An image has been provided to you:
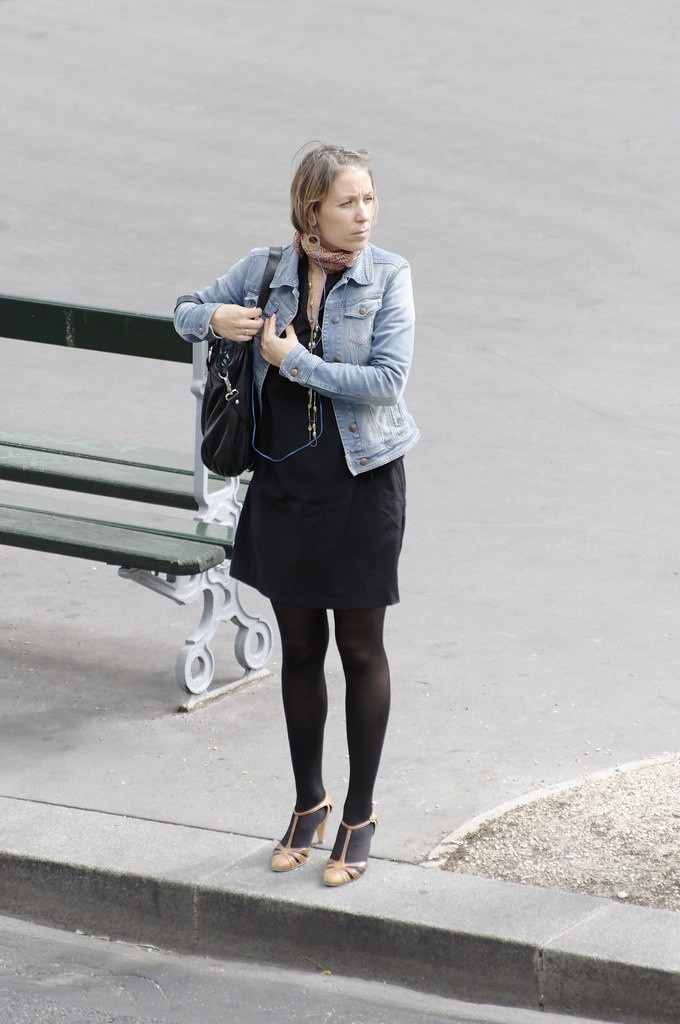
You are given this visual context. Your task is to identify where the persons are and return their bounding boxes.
[172,140,421,887]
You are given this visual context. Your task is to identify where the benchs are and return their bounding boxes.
[0,295,273,714]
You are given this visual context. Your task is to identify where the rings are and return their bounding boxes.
[246,329,248,336]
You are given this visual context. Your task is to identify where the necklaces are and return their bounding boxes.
[305,239,325,446]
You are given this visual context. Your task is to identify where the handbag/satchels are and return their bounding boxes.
[198,247,283,477]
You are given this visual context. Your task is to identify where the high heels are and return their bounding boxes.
[271,790,334,871]
[322,804,378,887]
